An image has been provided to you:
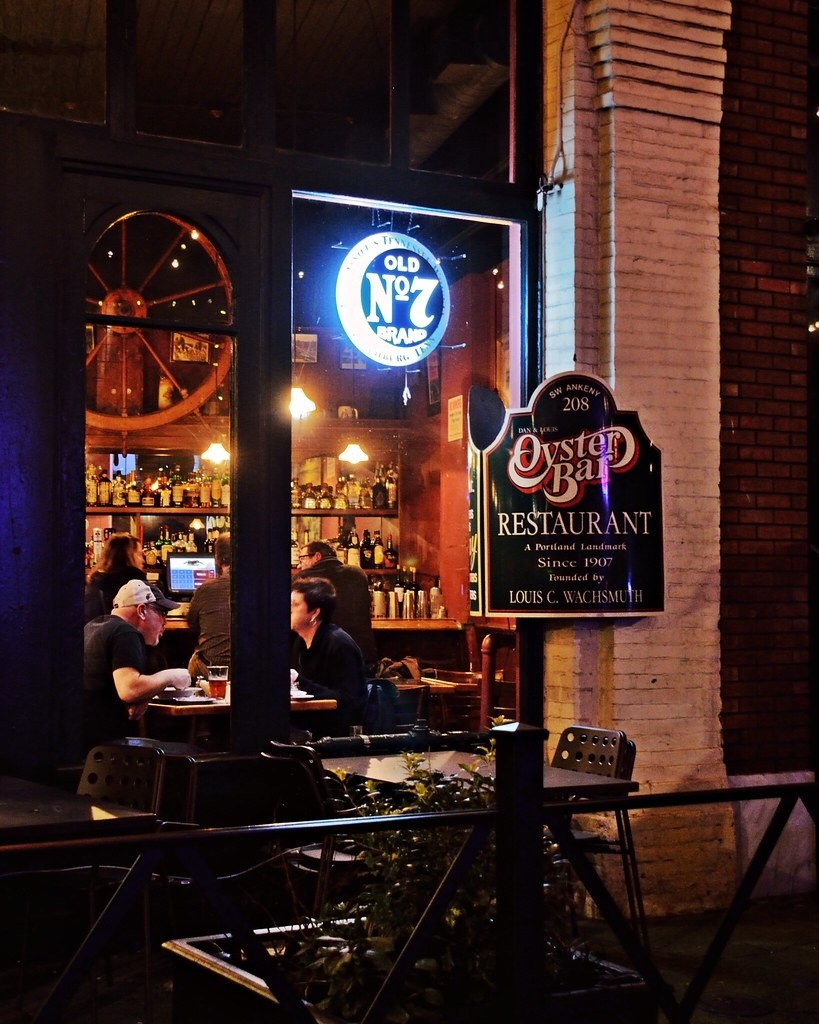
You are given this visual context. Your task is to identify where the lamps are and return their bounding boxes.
[338,346,369,465]
[200,252,230,465]
[290,291,315,418]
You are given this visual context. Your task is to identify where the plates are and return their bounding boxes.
[156,686,203,697]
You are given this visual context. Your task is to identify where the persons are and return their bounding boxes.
[291,577,367,740]
[181,531,231,681]
[293,541,371,662]
[84,579,191,738]
[84,533,145,625]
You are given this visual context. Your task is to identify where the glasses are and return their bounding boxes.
[299,554,316,564]
[147,604,168,617]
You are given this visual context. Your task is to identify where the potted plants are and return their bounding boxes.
[162,716,675,1024]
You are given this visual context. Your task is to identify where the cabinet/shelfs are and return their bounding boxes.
[86,492,400,576]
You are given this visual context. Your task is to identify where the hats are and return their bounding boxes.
[113,579,180,611]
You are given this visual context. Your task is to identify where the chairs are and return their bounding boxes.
[17,745,196,1024]
[257,740,364,921]
[549,727,650,956]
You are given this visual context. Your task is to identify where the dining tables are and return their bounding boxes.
[146,699,339,749]
[388,676,455,730]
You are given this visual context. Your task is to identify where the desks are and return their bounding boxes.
[0,777,157,1024]
[308,751,640,983]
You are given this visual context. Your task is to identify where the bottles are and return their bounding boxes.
[290,463,419,607]
[204,393,219,416]
[84,515,230,567]
[85,464,230,507]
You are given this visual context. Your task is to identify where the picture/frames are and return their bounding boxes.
[169,330,212,364]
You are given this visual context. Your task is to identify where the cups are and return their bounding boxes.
[207,665,229,699]
[338,405,359,419]
[371,587,444,618]
